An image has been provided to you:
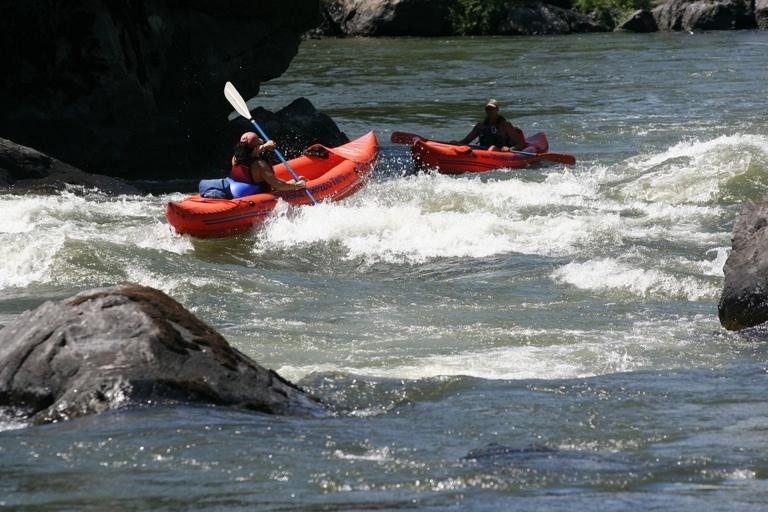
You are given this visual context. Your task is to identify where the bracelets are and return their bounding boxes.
[295,184,296,191]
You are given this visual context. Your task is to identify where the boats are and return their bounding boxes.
[410,131,548,174]
[166,130,379,238]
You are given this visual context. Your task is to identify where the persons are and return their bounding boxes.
[457,98,525,152]
[232,131,306,193]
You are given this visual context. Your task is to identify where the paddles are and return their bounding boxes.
[390,131,576,166]
[224,79,325,208]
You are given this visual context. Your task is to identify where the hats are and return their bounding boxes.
[485,98,497,108]
[240,132,263,146]
